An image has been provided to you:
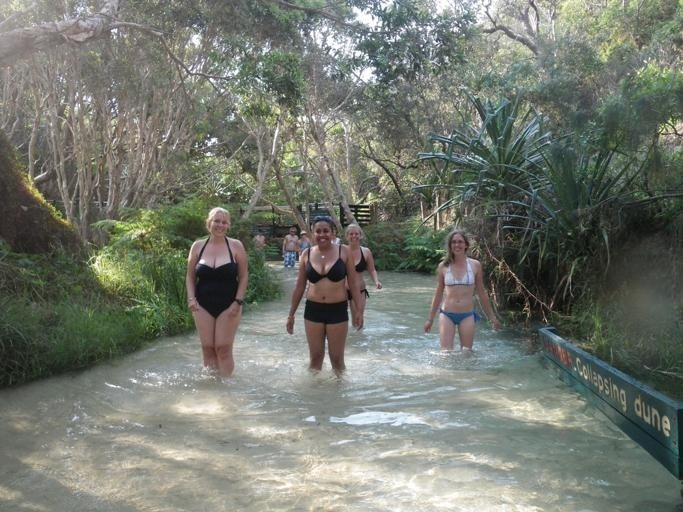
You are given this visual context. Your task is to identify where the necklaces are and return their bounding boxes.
[319,254,327,260]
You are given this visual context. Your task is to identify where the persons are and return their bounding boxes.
[183,205,249,380]
[296,229,311,261]
[281,226,300,269]
[330,229,340,246]
[422,227,502,359]
[285,213,363,382]
[251,229,266,262]
[340,222,382,332]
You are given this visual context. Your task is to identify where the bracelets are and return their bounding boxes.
[186,297,195,301]
[287,315,295,320]
[233,298,245,306]
[426,318,432,322]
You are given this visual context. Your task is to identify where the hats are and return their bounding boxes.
[300,230,307,235]
